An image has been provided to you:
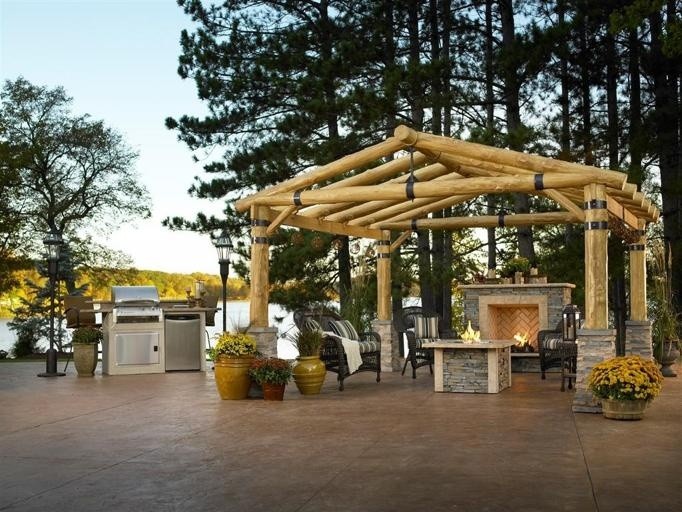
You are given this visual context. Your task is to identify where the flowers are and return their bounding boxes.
[245,356,295,388]
[206,330,262,362]
[584,353,666,404]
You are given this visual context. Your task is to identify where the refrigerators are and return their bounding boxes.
[162,314,202,373]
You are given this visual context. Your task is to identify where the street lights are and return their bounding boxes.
[212,224,234,371]
[38,222,67,377]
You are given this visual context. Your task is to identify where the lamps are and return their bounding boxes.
[559,303,582,342]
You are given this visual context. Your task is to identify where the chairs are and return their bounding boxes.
[536,302,586,380]
[293,306,382,391]
[394,304,459,380]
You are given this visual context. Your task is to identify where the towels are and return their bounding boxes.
[322,331,364,376]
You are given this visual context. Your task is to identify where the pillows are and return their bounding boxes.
[327,317,361,343]
[415,316,441,339]
[305,319,324,334]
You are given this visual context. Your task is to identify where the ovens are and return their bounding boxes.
[102,328,164,376]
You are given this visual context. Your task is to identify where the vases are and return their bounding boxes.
[212,352,253,401]
[260,381,284,401]
[597,397,647,420]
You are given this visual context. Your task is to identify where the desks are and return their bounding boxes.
[554,342,577,392]
[422,339,517,395]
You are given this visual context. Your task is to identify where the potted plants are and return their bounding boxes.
[286,326,328,395]
[70,326,103,377]
